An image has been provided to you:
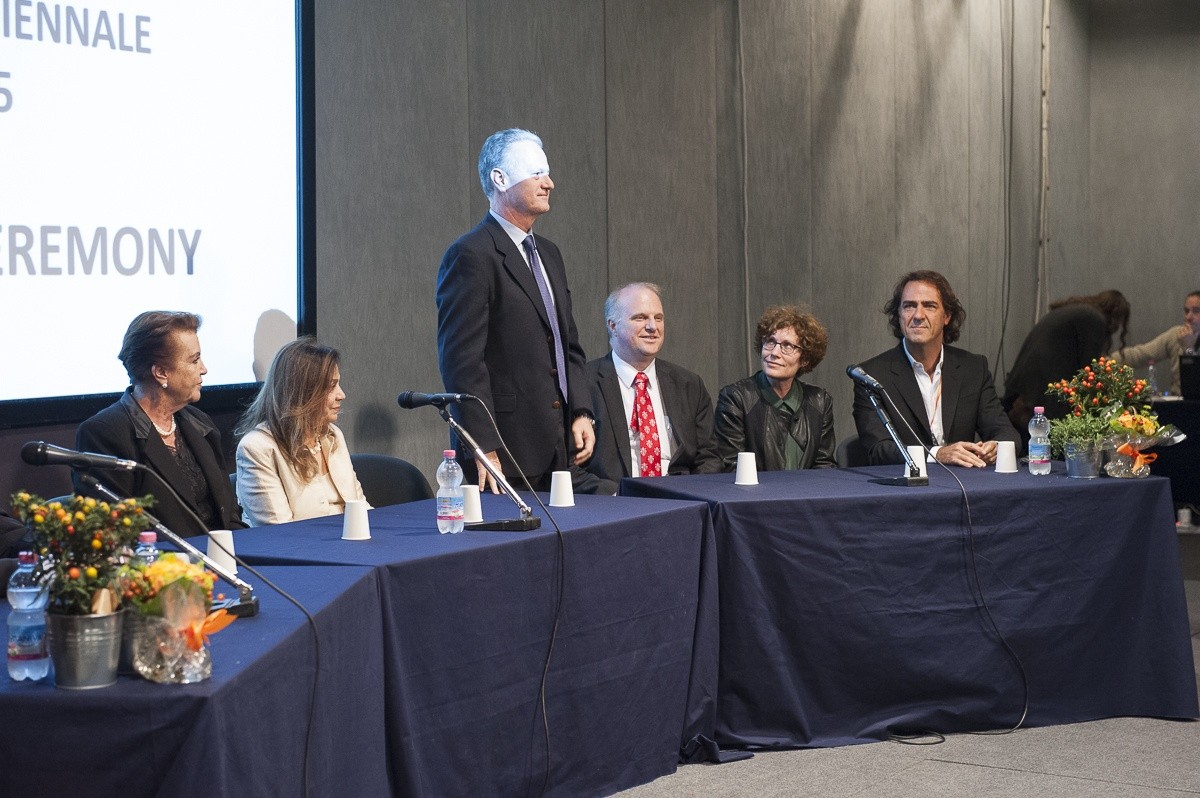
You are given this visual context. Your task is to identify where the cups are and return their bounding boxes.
[734,451,760,485]
[903,444,928,479]
[207,528,239,577]
[549,470,575,508]
[994,441,1019,474]
[459,483,484,524]
[341,499,371,540]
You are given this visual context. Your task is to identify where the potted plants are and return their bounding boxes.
[1089,404,1187,478]
[1046,414,1104,479]
[8,484,155,691]
[117,550,239,684]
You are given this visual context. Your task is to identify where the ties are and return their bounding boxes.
[522,234,570,406]
[629,373,664,478]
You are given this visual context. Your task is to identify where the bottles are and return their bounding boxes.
[1147,359,1159,396]
[5,547,51,682]
[135,531,158,572]
[436,449,464,535]
[1028,406,1052,475]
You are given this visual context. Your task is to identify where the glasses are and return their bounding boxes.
[761,336,803,355]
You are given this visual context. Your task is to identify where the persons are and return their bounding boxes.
[716,304,837,470]
[71,311,248,540]
[566,281,723,498]
[0,515,134,597]
[435,128,598,495]
[1004,290,1131,457]
[853,270,1022,469]
[235,336,375,528]
[1108,291,1199,396]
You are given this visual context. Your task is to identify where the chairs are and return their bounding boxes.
[350,452,434,511]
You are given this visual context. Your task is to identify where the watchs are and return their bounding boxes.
[574,414,597,431]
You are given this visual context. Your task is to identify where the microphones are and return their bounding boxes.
[20,440,146,473]
[846,364,884,391]
[398,390,474,409]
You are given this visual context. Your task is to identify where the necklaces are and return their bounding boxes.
[152,414,176,436]
[308,437,321,455]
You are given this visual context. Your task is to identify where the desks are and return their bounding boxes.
[156,482,716,798]
[0,560,390,798]
[619,457,1199,762]
